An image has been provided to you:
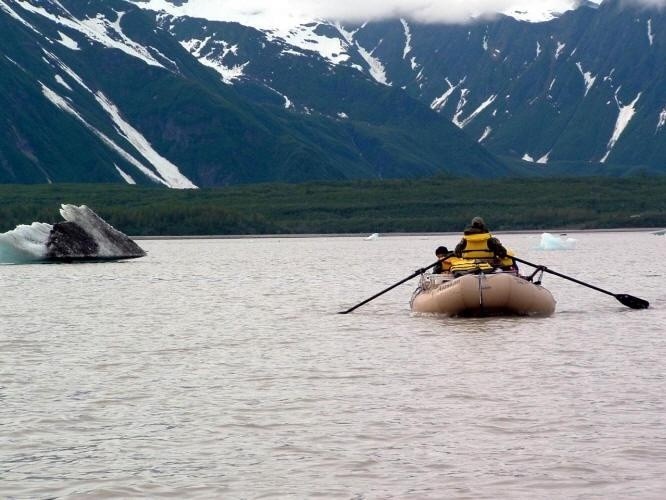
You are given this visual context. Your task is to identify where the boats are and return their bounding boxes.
[410,260,556,317]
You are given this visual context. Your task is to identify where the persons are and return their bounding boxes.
[432,216,506,274]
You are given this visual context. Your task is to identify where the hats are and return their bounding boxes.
[436,246,447,254]
[472,216,484,226]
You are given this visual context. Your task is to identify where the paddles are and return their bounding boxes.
[505,254,649,308]
